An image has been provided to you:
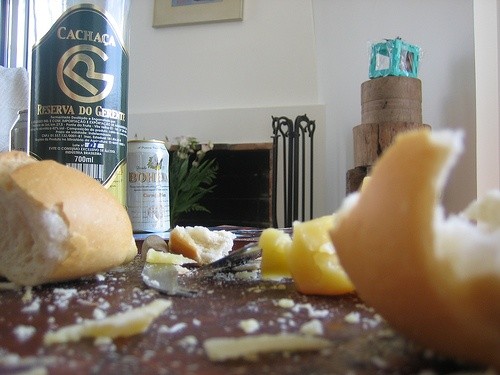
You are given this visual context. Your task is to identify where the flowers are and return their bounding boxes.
[164,136,221,232]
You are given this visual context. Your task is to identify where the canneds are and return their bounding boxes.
[127,139,171,234]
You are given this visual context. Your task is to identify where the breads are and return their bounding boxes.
[0,127,500,363]
